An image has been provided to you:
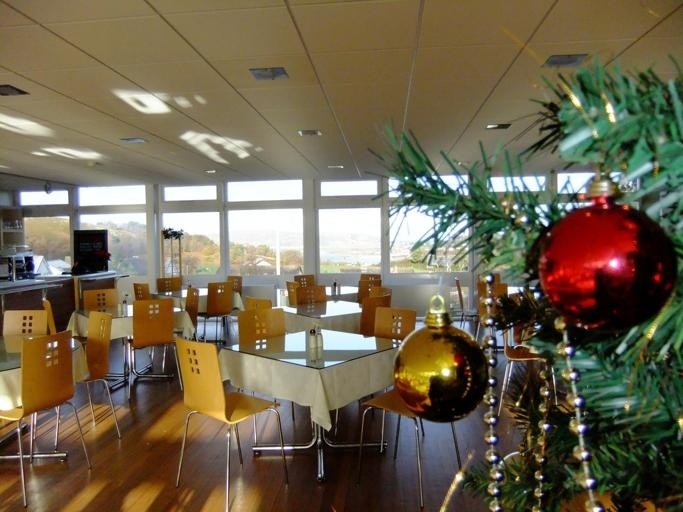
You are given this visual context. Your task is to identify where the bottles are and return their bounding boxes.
[124,293,130,308]
[3,218,23,229]
[187,280,192,288]
[330,279,341,287]
[309,349,325,369]
[331,287,341,293]
[310,326,323,348]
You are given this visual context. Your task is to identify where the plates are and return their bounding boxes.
[0,243,33,257]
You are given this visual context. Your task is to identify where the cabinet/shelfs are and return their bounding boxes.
[0,207,26,254]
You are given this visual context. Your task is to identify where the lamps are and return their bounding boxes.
[44,180,51,194]
